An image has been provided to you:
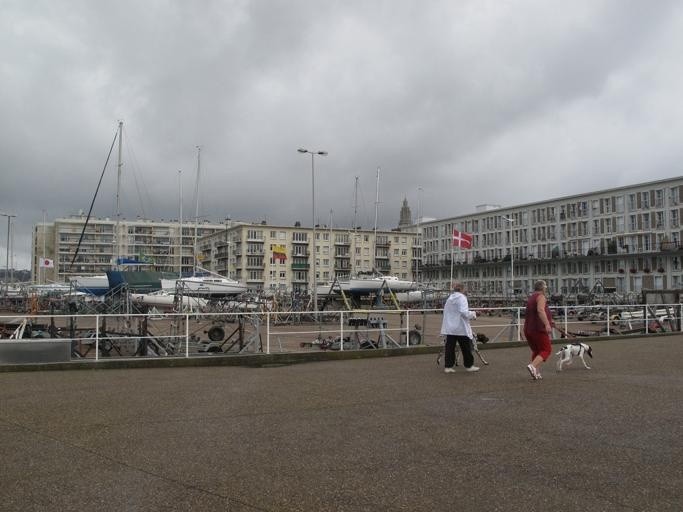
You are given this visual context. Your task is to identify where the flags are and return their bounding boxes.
[38,256,53,268]
[453,229,472,249]
[272,245,287,260]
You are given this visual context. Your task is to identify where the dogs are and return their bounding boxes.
[555,342,593,371]
[436,333,489,369]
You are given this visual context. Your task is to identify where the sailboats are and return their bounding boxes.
[312,165,448,303]
[1,122,256,308]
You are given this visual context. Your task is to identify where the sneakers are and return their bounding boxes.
[444,367,456,373]
[528,364,542,379]
[468,366,479,372]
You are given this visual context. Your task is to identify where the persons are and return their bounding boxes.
[523,280,553,380]
[439,282,481,373]
